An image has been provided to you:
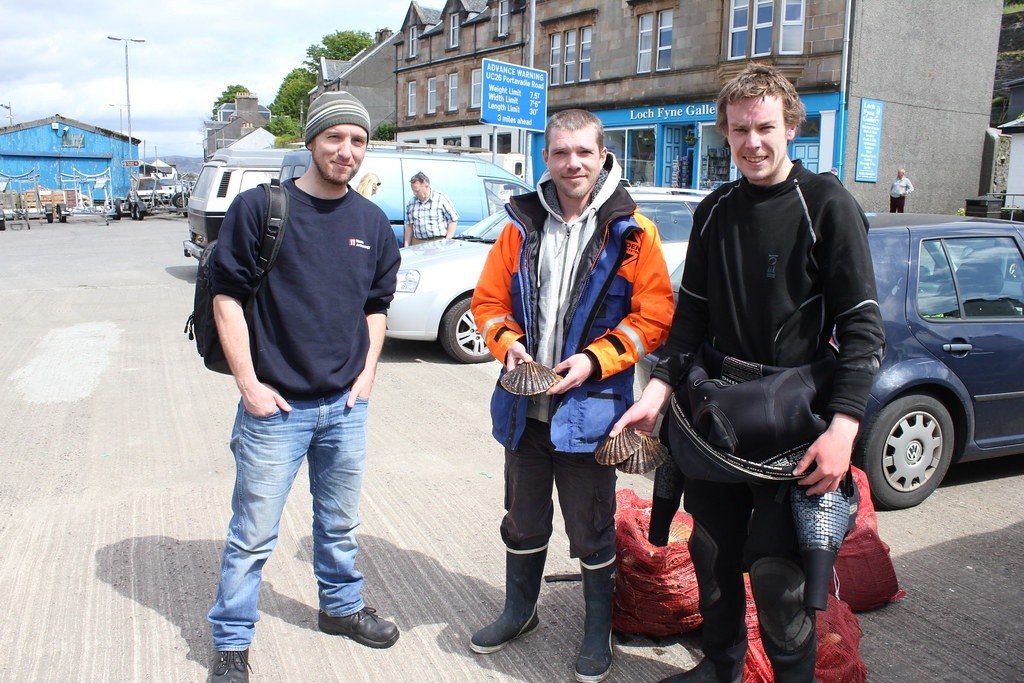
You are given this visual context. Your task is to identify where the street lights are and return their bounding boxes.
[107,36,145,192]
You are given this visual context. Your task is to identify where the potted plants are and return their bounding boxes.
[685,131,698,146]
[641,137,656,145]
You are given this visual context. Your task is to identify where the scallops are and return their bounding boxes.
[615,431,668,474]
[594,428,643,465]
[667,520,692,544]
[500,361,558,396]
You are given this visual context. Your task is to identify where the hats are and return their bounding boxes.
[304,91,371,147]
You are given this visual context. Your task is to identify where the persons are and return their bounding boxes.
[470,109,675,682]
[206,92,401,683]
[609,64,886,683]
[404,171,458,247]
[889,169,914,213]
[356,172,381,197]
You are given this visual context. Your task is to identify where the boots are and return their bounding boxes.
[469,542,548,654]
[574,554,617,683]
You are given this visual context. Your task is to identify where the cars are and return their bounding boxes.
[620,185,715,273]
[134,176,196,206]
[635,210,1023,512]
[383,208,502,365]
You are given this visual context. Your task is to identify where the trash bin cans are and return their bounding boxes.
[965,196,1003,219]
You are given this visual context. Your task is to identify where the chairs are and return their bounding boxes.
[920,262,1021,316]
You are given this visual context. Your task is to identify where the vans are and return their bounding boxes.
[272,140,538,249]
[181,142,304,262]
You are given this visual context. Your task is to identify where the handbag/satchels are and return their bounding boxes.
[183,178,289,376]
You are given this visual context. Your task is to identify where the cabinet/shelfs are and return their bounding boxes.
[705,145,730,182]
[671,153,690,188]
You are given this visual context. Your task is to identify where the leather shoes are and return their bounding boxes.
[211,648,253,683]
[319,605,400,649]
